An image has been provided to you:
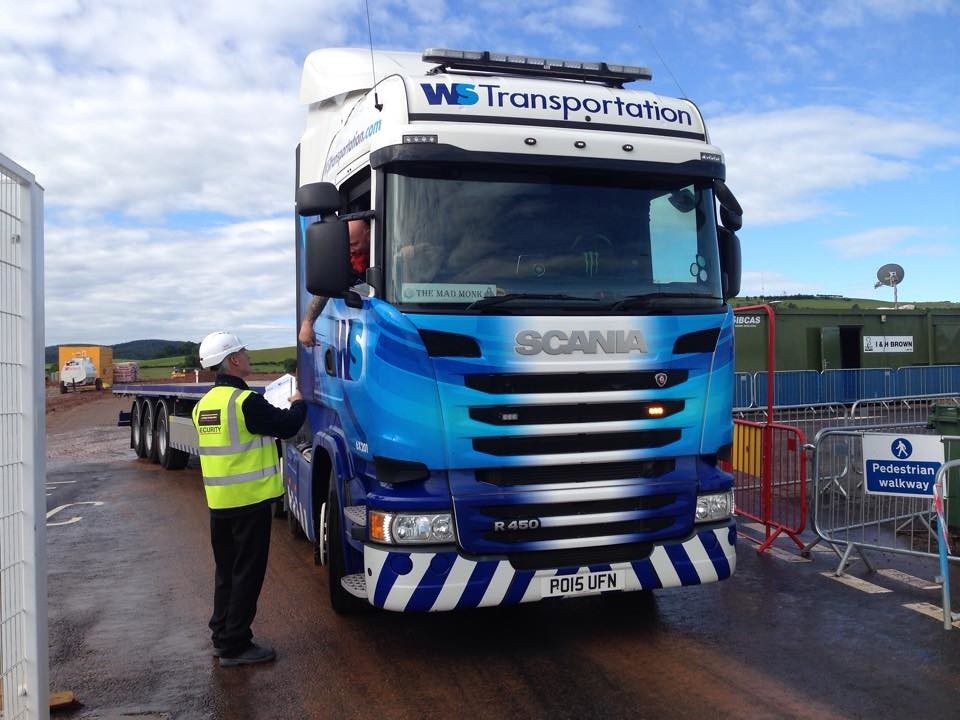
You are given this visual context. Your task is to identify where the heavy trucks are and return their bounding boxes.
[109,45,747,620]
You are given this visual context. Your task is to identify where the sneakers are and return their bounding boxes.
[213,645,227,656]
[219,646,276,666]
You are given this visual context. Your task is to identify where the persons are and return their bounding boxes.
[192,331,308,667]
[299,217,371,346]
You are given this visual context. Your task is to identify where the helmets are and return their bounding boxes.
[199,331,249,370]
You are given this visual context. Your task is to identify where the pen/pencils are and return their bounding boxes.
[290,377,292,396]
